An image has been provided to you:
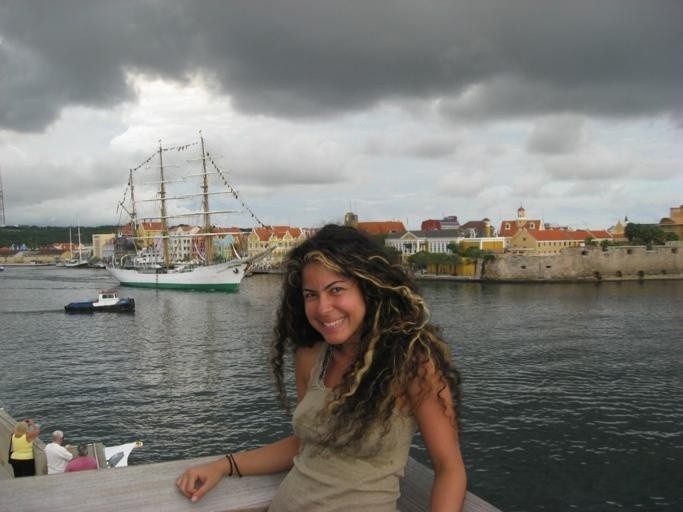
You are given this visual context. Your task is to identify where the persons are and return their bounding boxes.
[8,417,40,478]
[43,428,72,475]
[63,444,96,473]
[174,225,469,511]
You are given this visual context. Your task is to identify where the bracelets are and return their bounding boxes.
[224,450,242,479]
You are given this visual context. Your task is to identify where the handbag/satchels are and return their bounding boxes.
[8,433,13,463]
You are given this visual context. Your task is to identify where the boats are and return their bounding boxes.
[64,292,136,314]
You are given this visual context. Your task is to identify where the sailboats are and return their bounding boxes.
[105,136,279,292]
[64,225,89,267]
